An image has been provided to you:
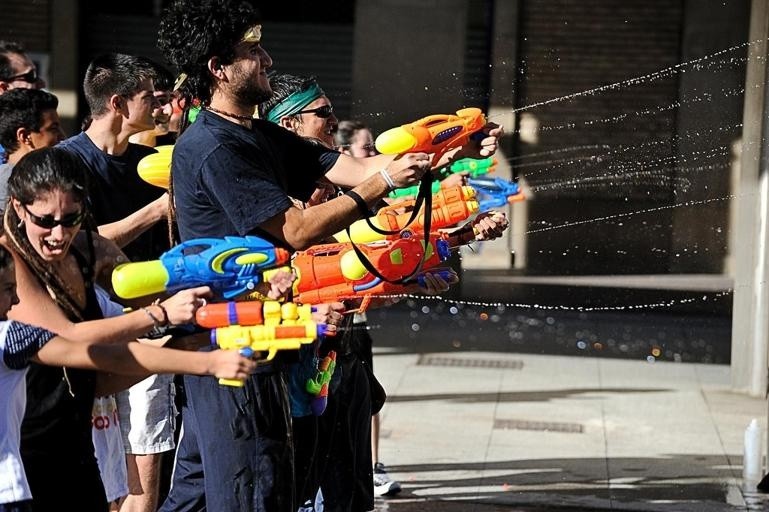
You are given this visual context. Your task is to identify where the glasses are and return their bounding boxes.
[4,70,37,83]
[290,105,333,118]
[20,203,86,228]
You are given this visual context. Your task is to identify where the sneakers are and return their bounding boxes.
[373,467,402,497]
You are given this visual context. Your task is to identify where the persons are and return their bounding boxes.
[0,38,511,511]
[149,3,507,511]
